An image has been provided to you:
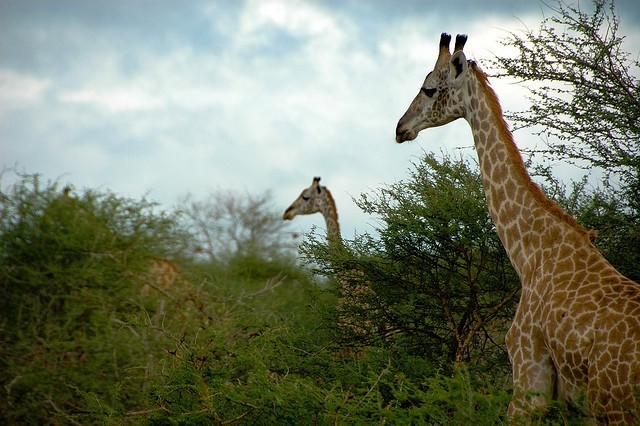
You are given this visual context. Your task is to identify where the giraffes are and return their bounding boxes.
[393,30,639,424]
[131,246,236,367]
[281,174,407,359]
